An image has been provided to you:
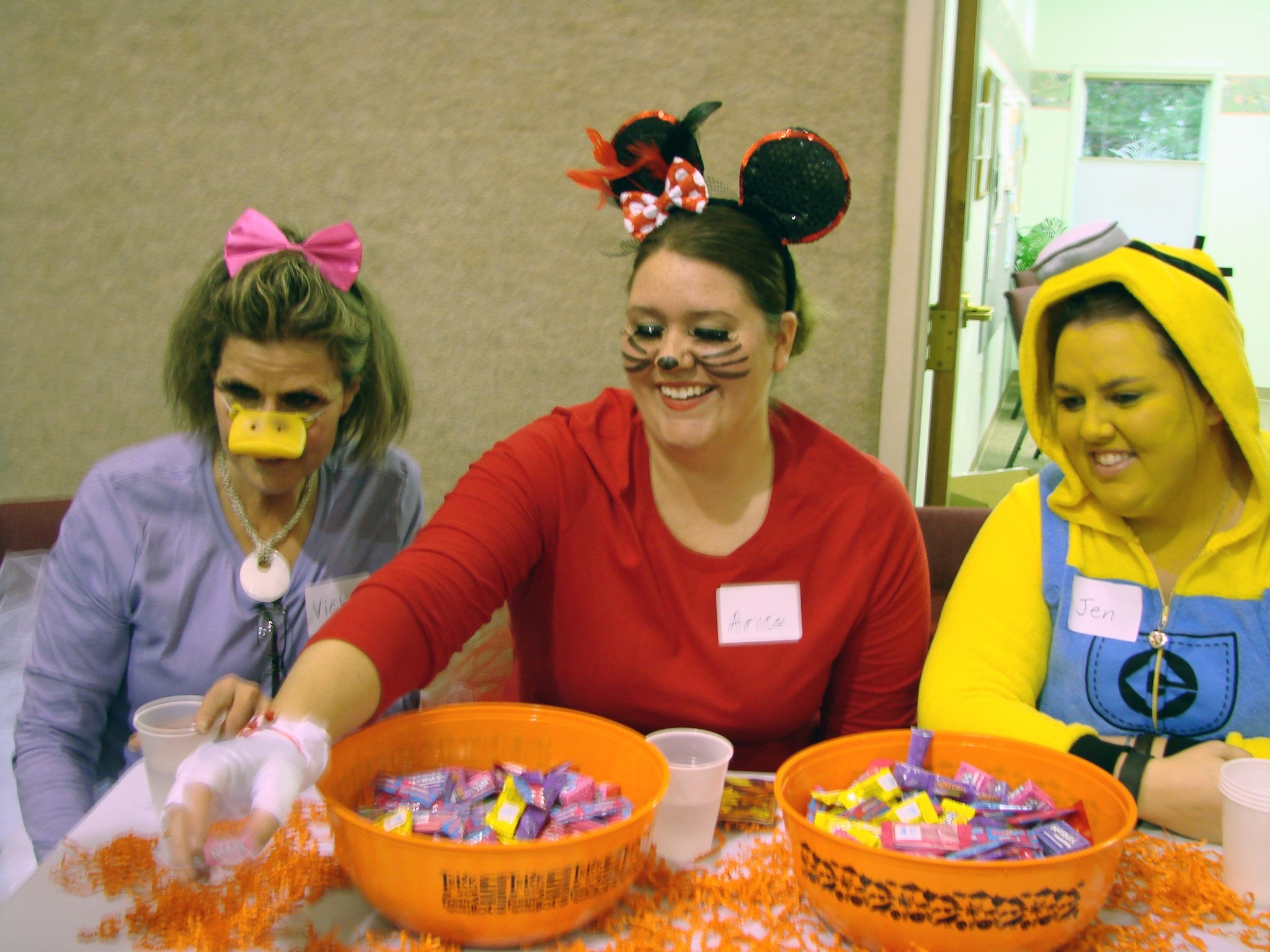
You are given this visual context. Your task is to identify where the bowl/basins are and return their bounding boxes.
[771,729,1140,952]
[313,699,670,946]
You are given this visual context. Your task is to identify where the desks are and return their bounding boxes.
[0,749,1267,952]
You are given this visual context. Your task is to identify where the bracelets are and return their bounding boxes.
[1115,752,1152,828]
[1126,733,1156,756]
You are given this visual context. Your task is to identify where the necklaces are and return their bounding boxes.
[1126,447,1234,598]
[217,446,316,602]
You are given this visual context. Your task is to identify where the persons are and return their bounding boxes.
[911,239,1268,845]
[11,209,421,864]
[165,202,932,894]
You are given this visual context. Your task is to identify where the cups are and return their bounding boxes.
[643,728,736,869]
[133,695,228,808]
[1215,754,1270,911]
